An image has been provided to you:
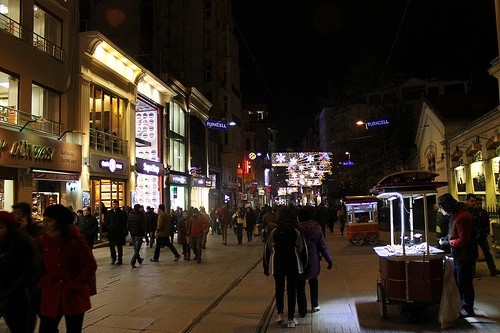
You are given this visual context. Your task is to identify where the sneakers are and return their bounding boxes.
[275,314,284,325]
[287,317,298,328]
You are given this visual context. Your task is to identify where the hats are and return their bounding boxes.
[43,204,74,224]
[437,193,458,213]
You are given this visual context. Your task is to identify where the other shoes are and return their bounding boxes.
[490,269,500,277]
[458,302,475,319]
[473,273,481,280]
[112,257,202,269]
[222,239,253,247]
[311,305,320,312]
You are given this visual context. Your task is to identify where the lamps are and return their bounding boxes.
[440,153,461,164]
[71,181,77,191]
[57,130,86,141]
[19,116,50,132]
[475,135,500,152]
[454,146,480,159]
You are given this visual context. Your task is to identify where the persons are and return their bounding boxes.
[263,208,309,327]
[178,211,190,261]
[217,204,231,245]
[245,207,257,242]
[186,208,207,263]
[32,204,98,333]
[437,192,478,319]
[232,207,246,245]
[128,204,148,268]
[295,207,333,318]
[122,204,222,249]
[227,204,238,235]
[66,204,98,252]
[254,202,347,246]
[105,200,128,265]
[0,202,42,333]
[150,204,181,262]
[99,202,108,241]
[440,194,500,277]
